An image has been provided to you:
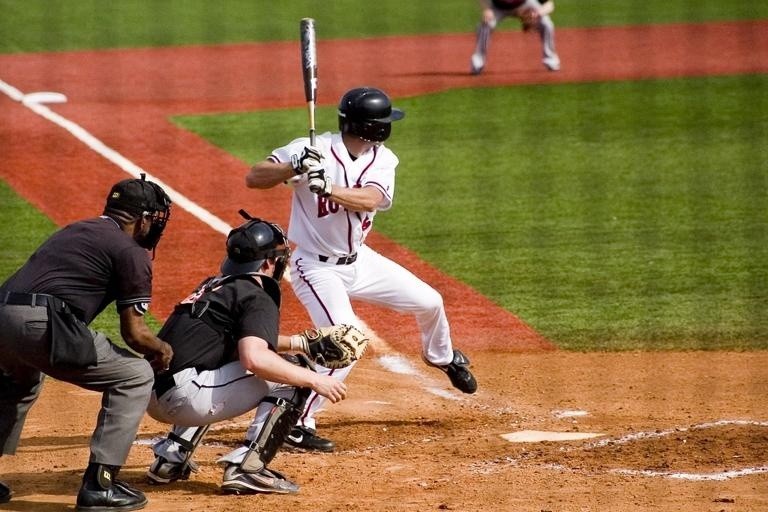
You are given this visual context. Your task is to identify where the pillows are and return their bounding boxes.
[106,179,170,250]
[338,87,404,142]
[219,220,288,282]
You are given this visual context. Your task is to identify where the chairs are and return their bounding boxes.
[281,423,337,453]
[420,348,478,394]
[148,457,188,483]
[221,462,300,493]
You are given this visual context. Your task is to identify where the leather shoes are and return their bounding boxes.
[292,147,331,197]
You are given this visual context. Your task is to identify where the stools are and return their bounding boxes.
[1,290,50,305]
[320,254,359,265]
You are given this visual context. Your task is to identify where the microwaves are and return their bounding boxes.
[301,16,323,192]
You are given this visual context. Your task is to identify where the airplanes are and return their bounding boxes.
[290,324,367,370]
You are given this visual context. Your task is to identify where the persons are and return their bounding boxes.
[244,88,477,452]
[0,179,174,512]
[470,1,561,74]
[142,217,369,495]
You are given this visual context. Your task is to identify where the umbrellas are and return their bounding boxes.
[75,464,148,512]
